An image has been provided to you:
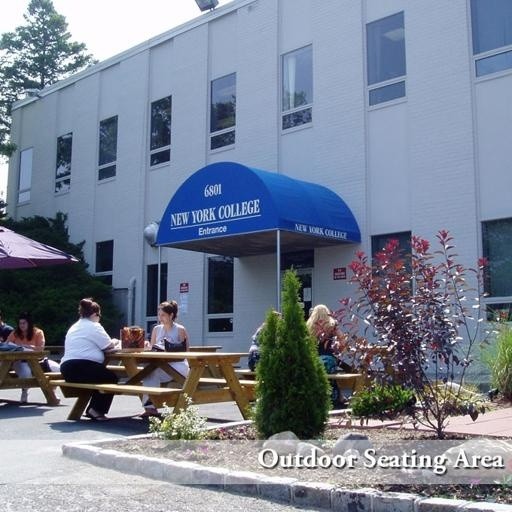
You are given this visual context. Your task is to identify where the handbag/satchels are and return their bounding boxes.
[120,326,146,352]
[163,336,187,352]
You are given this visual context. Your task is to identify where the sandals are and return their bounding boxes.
[87,409,113,424]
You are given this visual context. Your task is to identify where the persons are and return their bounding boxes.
[0,310,15,344]
[6,311,60,404]
[137,299,192,417]
[247,310,283,372]
[303,304,377,410]
[59,297,118,422]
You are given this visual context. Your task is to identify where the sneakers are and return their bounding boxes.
[21,392,28,404]
[140,411,158,418]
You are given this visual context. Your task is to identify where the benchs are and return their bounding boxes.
[0,346,394,420]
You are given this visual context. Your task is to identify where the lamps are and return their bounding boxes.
[195,0,219,12]
